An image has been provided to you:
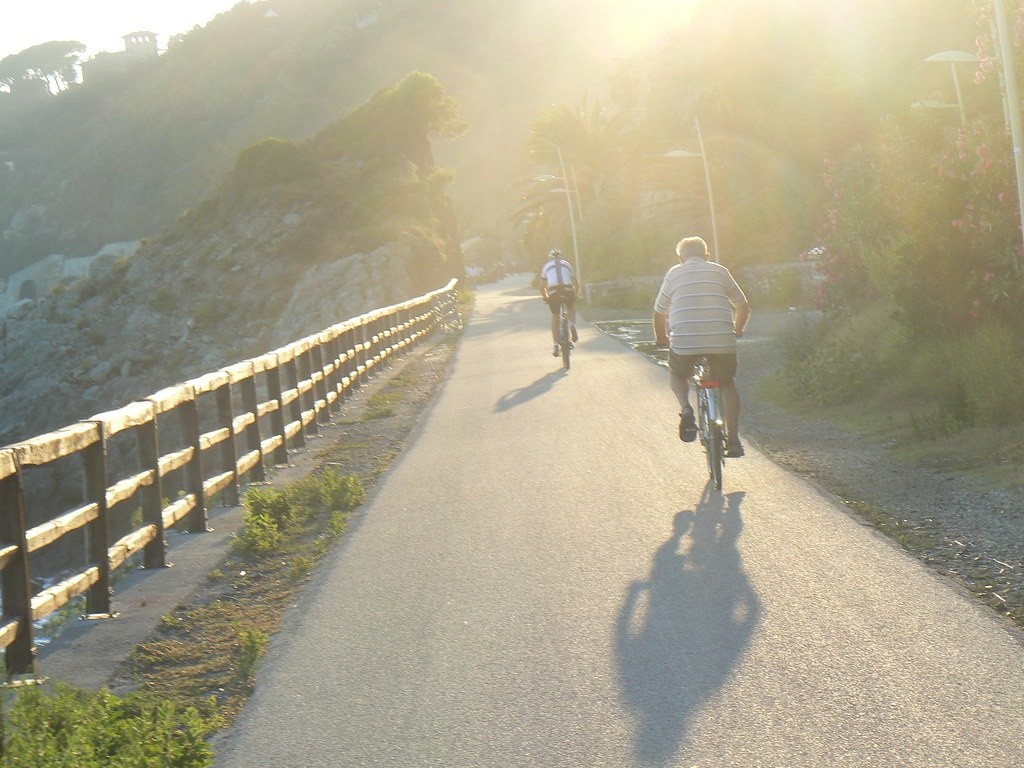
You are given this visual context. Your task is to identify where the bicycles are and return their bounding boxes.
[542,293,581,369]
[656,332,743,490]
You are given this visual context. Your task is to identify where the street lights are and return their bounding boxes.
[660,115,719,264]
[533,147,584,303]
[907,49,1023,221]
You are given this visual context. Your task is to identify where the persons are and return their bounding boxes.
[540,249,580,357]
[653,236,751,458]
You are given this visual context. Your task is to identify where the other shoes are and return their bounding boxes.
[571,325,578,341]
[680,413,696,442]
[553,345,559,356]
[726,440,744,457]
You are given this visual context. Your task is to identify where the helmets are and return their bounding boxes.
[549,249,561,257]
